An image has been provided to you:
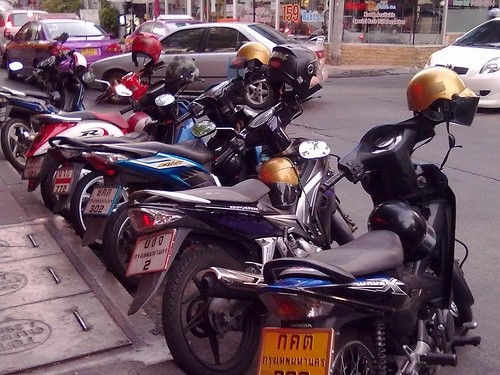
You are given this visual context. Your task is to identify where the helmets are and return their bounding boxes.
[165,56,200,91]
[259,157,301,207]
[56,57,74,73]
[407,66,480,126]
[271,43,318,98]
[227,42,271,79]
[131,32,162,67]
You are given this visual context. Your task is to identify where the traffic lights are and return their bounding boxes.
[117,14,126,26]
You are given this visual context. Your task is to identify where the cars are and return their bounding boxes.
[5,19,123,81]
[424,15,500,110]
[84,23,328,105]
[25,13,79,20]
[124,13,196,53]
[0,9,48,69]
[306,28,366,43]
[487,7,500,19]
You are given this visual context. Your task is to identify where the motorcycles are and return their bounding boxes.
[0,32,94,171]
[126,83,359,375]
[18,60,322,292]
[255,99,481,375]
